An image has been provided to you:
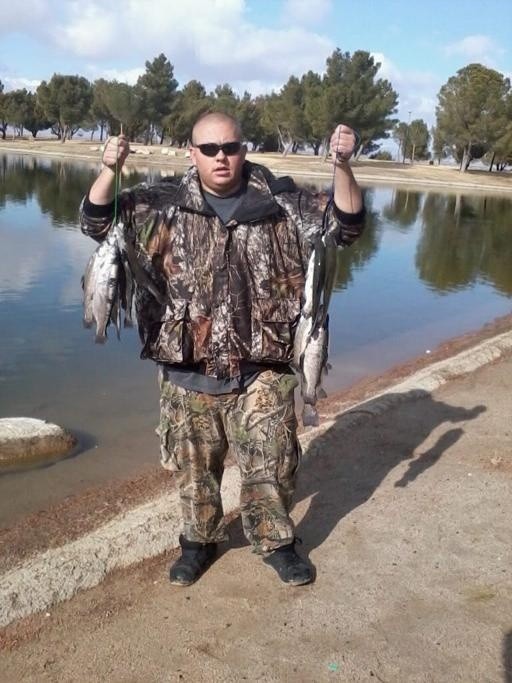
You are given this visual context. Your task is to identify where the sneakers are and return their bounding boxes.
[170,534,217,587]
[263,537,312,586]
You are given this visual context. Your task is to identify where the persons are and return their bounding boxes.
[76,107,365,588]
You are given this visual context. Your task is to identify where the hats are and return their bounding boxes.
[194,142,241,156]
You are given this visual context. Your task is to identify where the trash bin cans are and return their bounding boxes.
[430,161,433,164]
